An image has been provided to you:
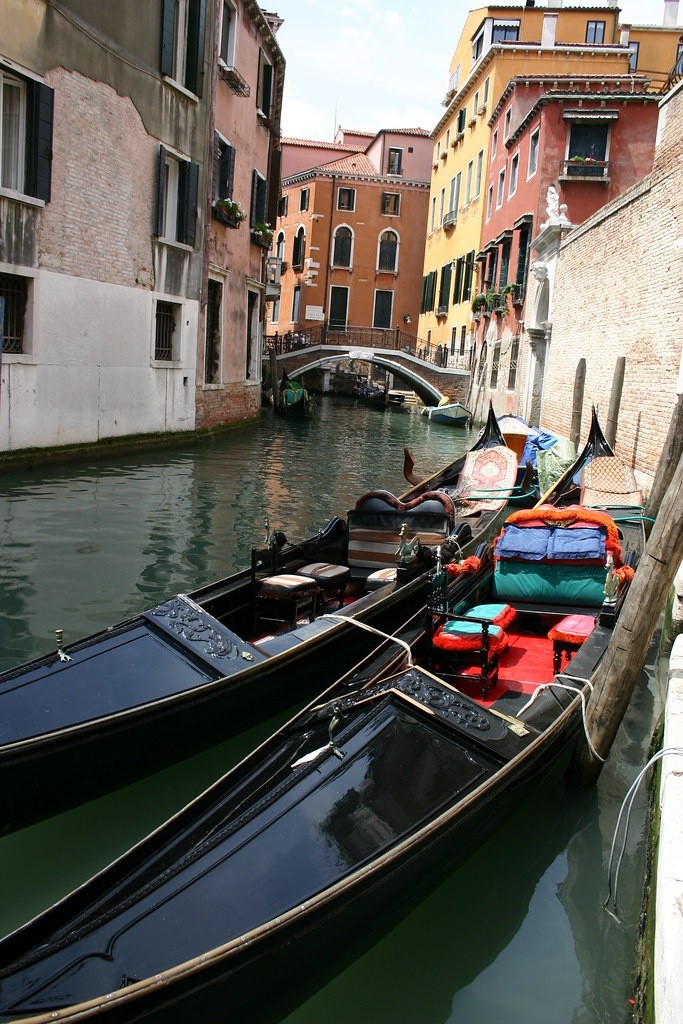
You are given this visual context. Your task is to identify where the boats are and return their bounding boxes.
[0,405,654,1024]
[358,379,390,405]
[428,401,472,426]
[387,390,407,407]
[0,398,519,774]
[279,366,314,421]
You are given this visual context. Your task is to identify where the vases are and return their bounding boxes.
[218,206,228,215]
[584,161,594,165]
[257,234,268,241]
[596,161,607,166]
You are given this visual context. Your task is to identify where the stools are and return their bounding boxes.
[365,568,396,595]
[547,613,598,676]
[295,561,352,616]
[462,602,518,654]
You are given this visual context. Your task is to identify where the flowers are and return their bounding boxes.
[254,222,273,243]
[585,158,597,162]
[212,197,248,222]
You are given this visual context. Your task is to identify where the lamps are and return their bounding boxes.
[404,315,412,325]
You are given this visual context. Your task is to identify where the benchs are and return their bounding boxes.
[344,509,451,585]
[483,509,633,634]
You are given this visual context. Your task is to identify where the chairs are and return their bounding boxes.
[249,544,319,633]
[426,565,510,701]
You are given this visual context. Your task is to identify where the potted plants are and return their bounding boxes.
[569,156,585,166]
[472,283,519,318]
